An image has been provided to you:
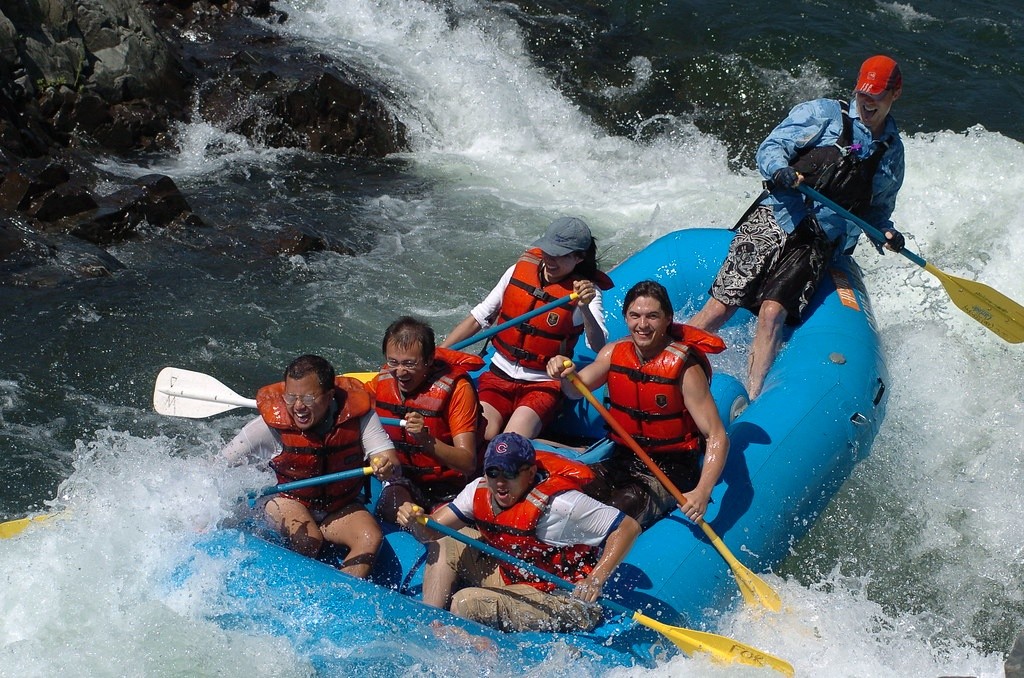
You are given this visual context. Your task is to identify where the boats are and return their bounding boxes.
[176,228,889,677]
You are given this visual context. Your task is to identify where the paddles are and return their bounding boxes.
[1,457,381,544]
[410,503,795,678]
[560,359,783,612]
[795,181,1023,344]
[153,366,407,428]
[336,287,580,383]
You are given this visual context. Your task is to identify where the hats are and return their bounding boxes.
[484,433,537,470]
[532,216,591,256]
[853,57,902,100]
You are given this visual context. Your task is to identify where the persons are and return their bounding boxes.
[436,216,615,442]
[546,280,729,537]
[364,316,479,530]
[396,430,644,634]
[220,355,404,580]
[685,56,907,402]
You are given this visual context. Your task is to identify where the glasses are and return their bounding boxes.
[387,359,426,368]
[282,392,323,406]
[485,466,528,480]
